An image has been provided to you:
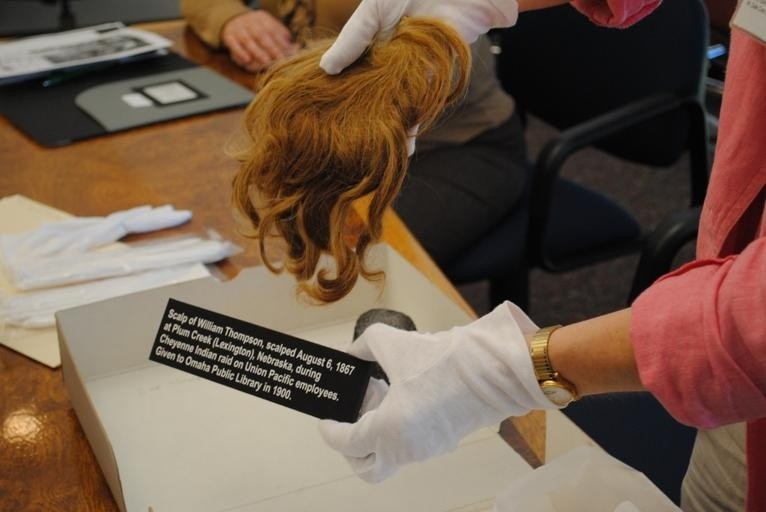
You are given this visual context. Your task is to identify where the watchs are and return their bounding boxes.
[531,324,578,408]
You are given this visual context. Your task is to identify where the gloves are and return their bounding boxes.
[318,0,519,75]
[316,300,559,487]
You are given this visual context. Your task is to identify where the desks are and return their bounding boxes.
[0,14,602,511]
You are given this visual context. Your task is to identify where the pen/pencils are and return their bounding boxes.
[42,68,95,87]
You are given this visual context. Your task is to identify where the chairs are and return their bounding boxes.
[625,206,700,304]
[470,0,710,314]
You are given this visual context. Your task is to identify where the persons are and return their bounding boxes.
[181,0,523,270]
[319,0,765,512]
